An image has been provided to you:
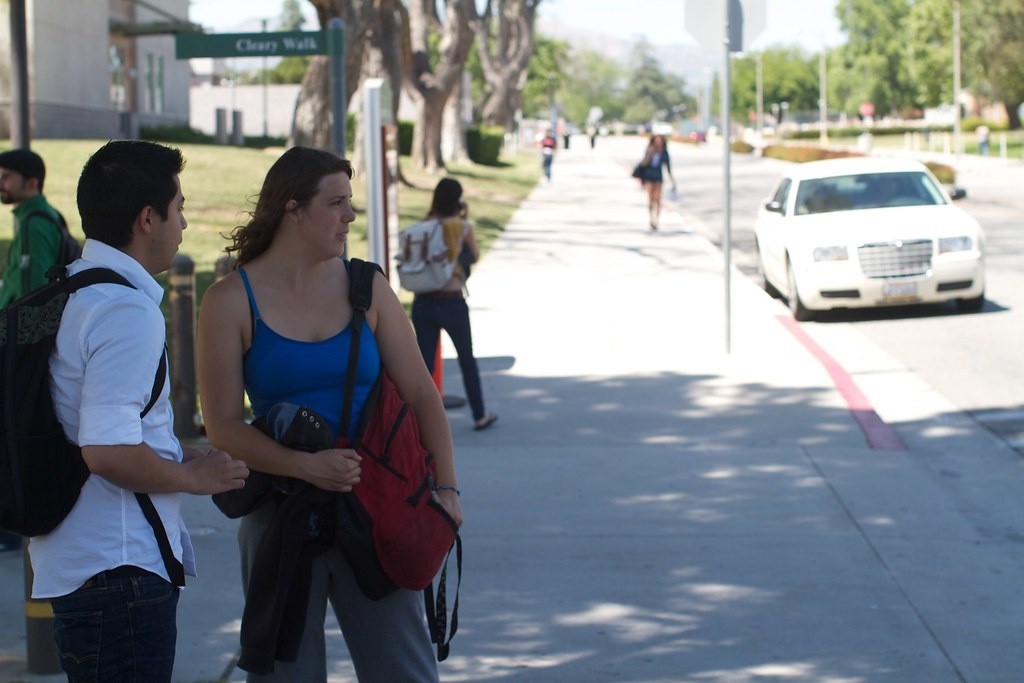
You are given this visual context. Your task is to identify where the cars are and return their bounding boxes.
[755,156,989,323]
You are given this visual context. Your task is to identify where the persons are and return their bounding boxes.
[198,147,464,683]
[411,177,499,432]
[877,178,898,203]
[975,125,990,157]
[642,136,676,232]
[0,139,249,683]
[541,128,556,180]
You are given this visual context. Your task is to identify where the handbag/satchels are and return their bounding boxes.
[633,163,648,179]
[332,257,451,591]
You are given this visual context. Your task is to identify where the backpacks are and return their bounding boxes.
[20,209,82,296]
[0,267,167,537]
[394,218,468,293]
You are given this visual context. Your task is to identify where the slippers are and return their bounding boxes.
[473,412,497,431]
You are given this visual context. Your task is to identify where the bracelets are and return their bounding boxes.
[432,484,460,497]
